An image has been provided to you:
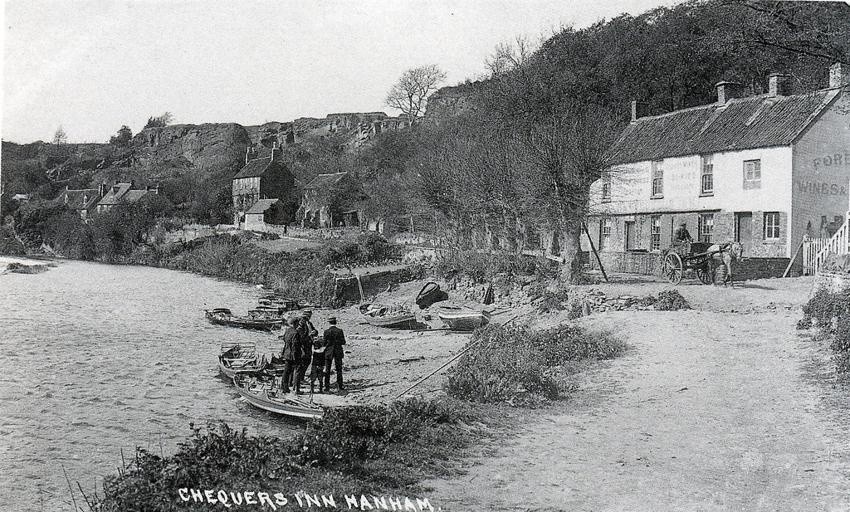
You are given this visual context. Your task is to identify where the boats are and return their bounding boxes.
[206,293,342,426]
[357,301,418,332]
[436,310,493,331]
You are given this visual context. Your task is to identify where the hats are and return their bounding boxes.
[327,317,337,322]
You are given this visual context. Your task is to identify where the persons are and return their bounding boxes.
[319,316,347,391]
[672,223,695,248]
[308,339,325,394]
[288,314,311,391]
[298,309,319,385]
[281,316,305,395]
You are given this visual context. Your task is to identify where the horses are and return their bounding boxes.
[707,242,745,290]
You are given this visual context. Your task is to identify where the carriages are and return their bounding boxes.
[660,240,746,287]
